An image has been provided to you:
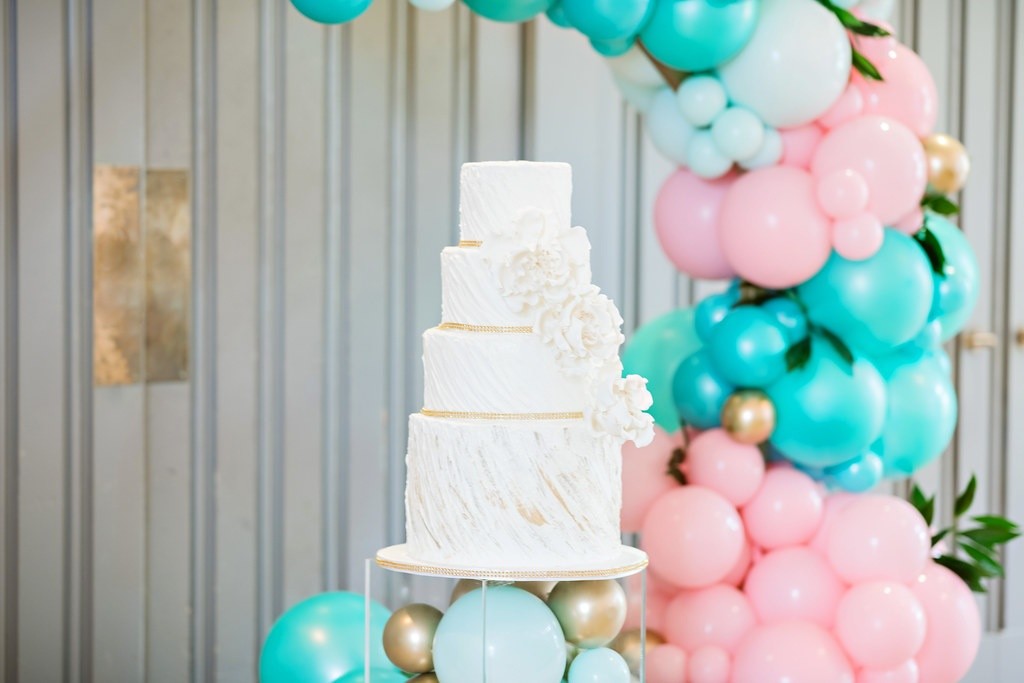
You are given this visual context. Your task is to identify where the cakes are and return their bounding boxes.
[403,159,656,567]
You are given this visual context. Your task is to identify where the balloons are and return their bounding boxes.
[259,0,983,682]
